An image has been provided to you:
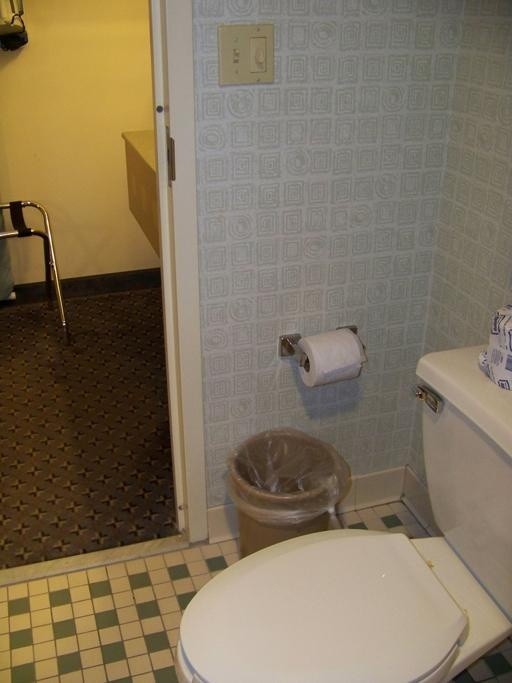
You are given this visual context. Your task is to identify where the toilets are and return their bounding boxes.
[178,345,512,682]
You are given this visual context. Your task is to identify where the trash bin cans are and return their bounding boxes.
[230,430,342,560]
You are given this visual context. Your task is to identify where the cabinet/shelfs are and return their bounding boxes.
[124,141,160,260]
